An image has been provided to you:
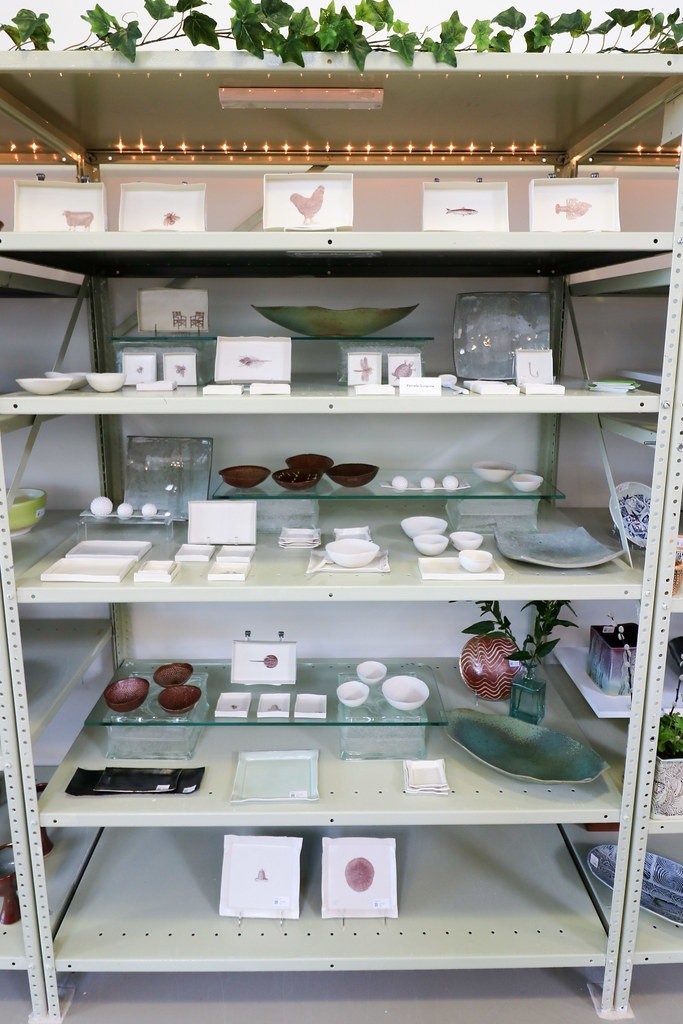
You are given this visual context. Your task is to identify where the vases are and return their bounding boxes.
[653,753,683,819]
[510,671,546,724]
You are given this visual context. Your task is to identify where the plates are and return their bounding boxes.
[229,748,320,802]
[587,844,683,926]
[442,708,611,785]
[552,648,683,719]
[493,526,625,568]
[401,758,450,796]
[608,481,652,547]
[65,766,206,795]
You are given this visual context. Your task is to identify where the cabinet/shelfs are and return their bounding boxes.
[1,42,682,1020]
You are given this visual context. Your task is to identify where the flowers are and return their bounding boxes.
[606,609,683,759]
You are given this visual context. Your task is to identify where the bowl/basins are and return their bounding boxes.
[218,453,379,488]
[251,304,419,337]
[472,461,544,492]
[326,515,493,573]
[103,662,202,713]
[335,661,430,710]
[7,487,47,537]
[15,370,128,393]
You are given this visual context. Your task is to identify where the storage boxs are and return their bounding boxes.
[263,172,354,231]
[420,180,510,233]
[119,182,211,232]
[342,343,565,395]
[213,640,328,718]
[12,178,107,230]
[123,288,293,394]
[527,176,622,232]
[41,500,256,583]
